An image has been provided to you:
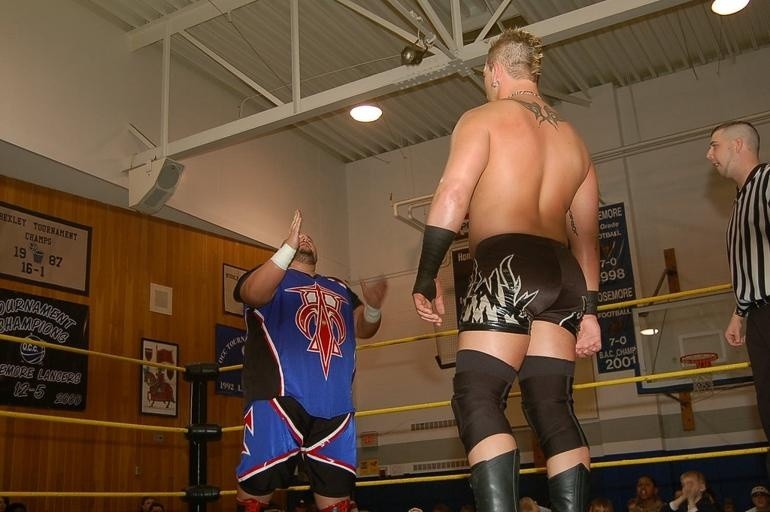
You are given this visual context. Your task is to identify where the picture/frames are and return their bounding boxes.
[0,201,92,298]
[139,337,181,418]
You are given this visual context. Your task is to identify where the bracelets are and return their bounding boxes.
[584,291,599,314]
[364,303,383,323]
[270,243,297,271]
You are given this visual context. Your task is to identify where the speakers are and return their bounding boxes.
[128,156,184,216]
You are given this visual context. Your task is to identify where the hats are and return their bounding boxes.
[749,482,769,496]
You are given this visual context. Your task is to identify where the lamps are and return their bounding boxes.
[349,101,384,124]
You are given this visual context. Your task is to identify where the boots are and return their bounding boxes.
[545,462,592,512]
[469,449,521,512]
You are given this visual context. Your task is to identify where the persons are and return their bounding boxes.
[587,472,770,512]
[413,26,603,512]
[705,121,770,441]
[0,497,26,512]
[519,497,552,512]
[233,208,387,512]
[294,498,308,512]
[141,497,165,512]
